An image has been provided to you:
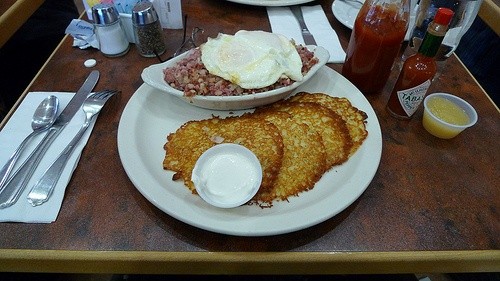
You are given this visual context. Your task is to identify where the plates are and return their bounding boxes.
[117,64,383,236]
[332,0,419,40]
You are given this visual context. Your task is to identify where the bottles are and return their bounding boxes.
[133,2,168,57]
[341,0,411,95]
[384,7,454,121]
[399,0,469,81]
[91,3,130,57]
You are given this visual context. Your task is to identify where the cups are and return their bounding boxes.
[422,93,478,139]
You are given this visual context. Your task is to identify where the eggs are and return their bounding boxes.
[200,32,285,89]
[234,30,303,82]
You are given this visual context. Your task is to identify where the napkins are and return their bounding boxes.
[0,91,100,224]
[266,5,348,64]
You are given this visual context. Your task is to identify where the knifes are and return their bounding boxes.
[0,70,100,209]
[290,5,316,45]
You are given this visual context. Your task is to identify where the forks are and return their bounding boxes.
[26,89,121,207]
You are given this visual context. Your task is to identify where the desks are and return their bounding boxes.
[0,0,500,281]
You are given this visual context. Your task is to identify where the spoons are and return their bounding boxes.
[0,95,59,190]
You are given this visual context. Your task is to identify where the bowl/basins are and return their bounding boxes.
[140,44,329,110]
[193,144,263,209]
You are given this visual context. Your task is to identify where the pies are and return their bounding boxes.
[163,91,369,205]
